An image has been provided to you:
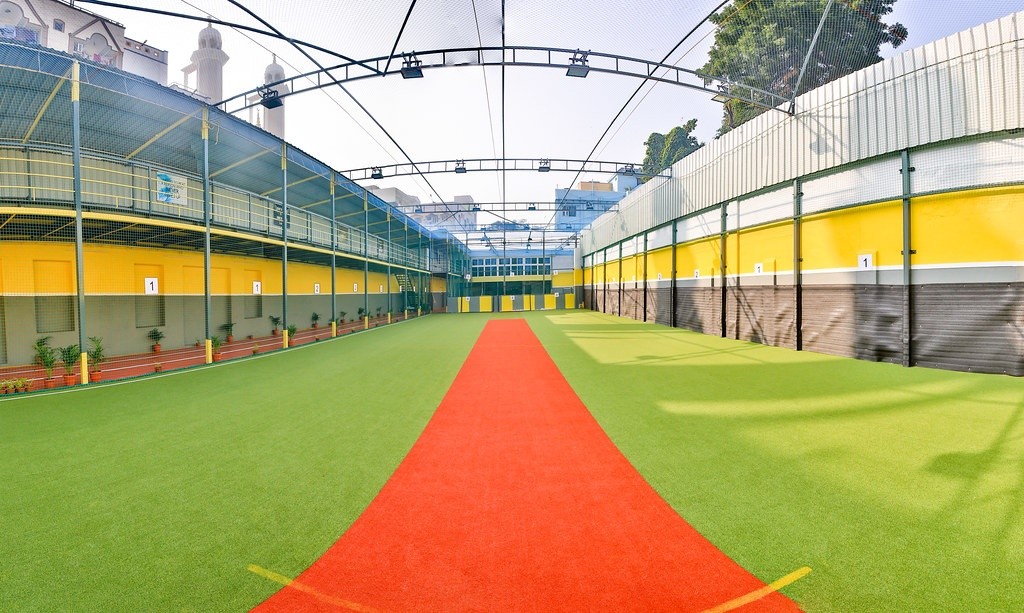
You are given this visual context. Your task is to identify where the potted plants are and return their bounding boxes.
[147,307,382,372]
[0,336,103,395]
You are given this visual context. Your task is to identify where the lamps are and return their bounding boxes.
[401,61,423,79]
[455,163,466,173]
[371,169,383,179]
[260,90,283,109]
[566,58,590,78]
[415,206,421,212]
[528,204,535,210]
[623,166,634,176]
[538,162,549,172]
[711,94,731,103]
[473,204,480,210]
